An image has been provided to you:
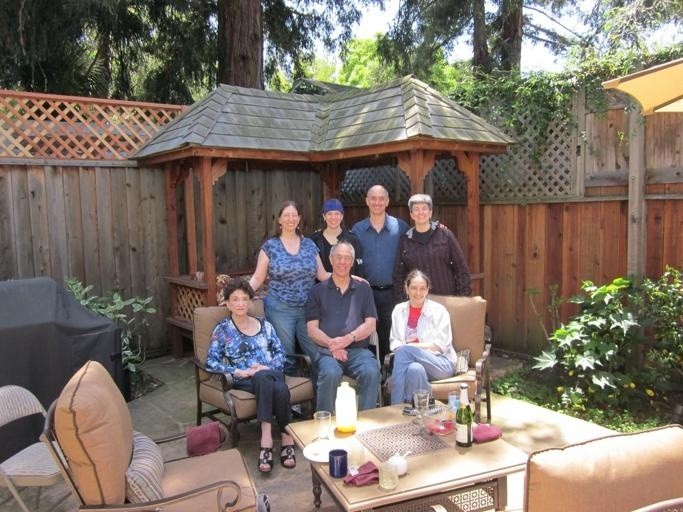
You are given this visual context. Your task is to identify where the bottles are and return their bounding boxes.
[455,383,473,447]
[334,381,357,433]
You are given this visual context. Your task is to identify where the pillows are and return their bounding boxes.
[194,271,230,306]
[455,347,470,375]
[125,428,165,506]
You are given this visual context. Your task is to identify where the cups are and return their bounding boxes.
[414,390,430,421]
[313,411,332,439]
[379,462,398,489]
[447,391,461,414]
[329,448,347,478]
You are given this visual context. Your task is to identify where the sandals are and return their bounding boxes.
[258,445,273,474]
[280,444,296,470]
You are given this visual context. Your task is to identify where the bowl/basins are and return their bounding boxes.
[421,404,456,435]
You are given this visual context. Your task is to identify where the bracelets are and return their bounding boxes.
[350,333,356,341]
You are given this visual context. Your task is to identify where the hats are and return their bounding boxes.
[322,198,345,216]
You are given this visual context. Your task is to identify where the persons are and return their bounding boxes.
[306,242,382,417]
[312,199,365,277]
[393,194,472,303]
[390,270,457,404]
[250,203,371,418]
[207,279,298,472]
[354,184,448,384]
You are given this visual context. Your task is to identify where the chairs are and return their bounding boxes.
[383,296,492,424]
[0,385,85,511]
[39,359,270,511]
[193,300,316,448]
[525,423,683,511]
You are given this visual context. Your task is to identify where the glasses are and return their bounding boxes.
[411,206,432,213]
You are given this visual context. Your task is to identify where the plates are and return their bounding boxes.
[303,441,337,463]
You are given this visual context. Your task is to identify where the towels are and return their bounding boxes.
[342,461,381,486]
[184,421,222,457]
[472,423,503,443]
